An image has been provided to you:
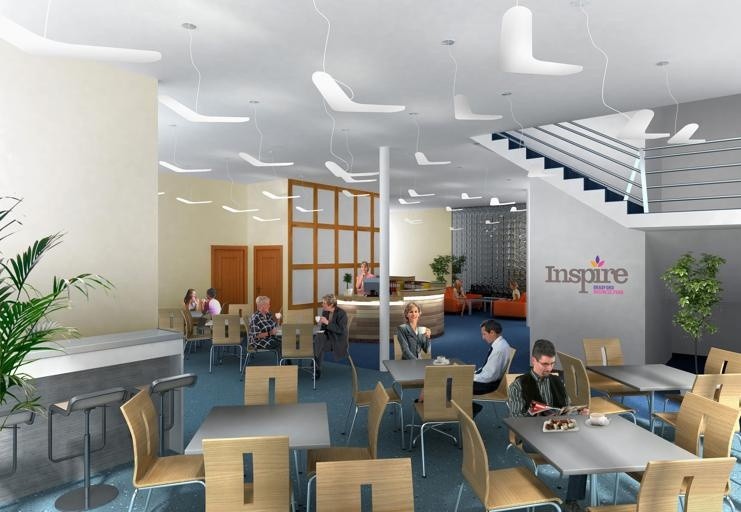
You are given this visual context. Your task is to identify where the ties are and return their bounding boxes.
[475,348,492,374]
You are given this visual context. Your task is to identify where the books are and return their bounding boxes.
[526,399,587,416]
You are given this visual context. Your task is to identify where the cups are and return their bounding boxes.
[315,316,322,323]
[590,413,604,425]
[274,313,283,320]
[418,327,426,335]
[436,356,444,364]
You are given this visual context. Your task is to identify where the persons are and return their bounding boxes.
[355,262,376,297]
[198,288,222,320]
[184,288,200,312]
[453,318,513,419]
[509,280,520,301]
[397,302,432,361]
[453,279,470,317]
[248,296,291,365]
[312,294,349,381]
[507,338,592,512]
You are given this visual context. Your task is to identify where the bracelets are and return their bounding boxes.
[267,331,271,336]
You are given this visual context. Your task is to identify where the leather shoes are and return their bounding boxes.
[561,500,582,511]
[472,404,483,419]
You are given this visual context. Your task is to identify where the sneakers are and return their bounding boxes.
[313,373,320,380]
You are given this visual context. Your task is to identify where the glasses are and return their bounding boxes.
[539,360,555,366]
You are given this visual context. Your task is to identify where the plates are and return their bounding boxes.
[544,419,579,432]
[584,418,609,428]
[433,360,449,366]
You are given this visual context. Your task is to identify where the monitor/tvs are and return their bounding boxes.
[362,277,382,297]
